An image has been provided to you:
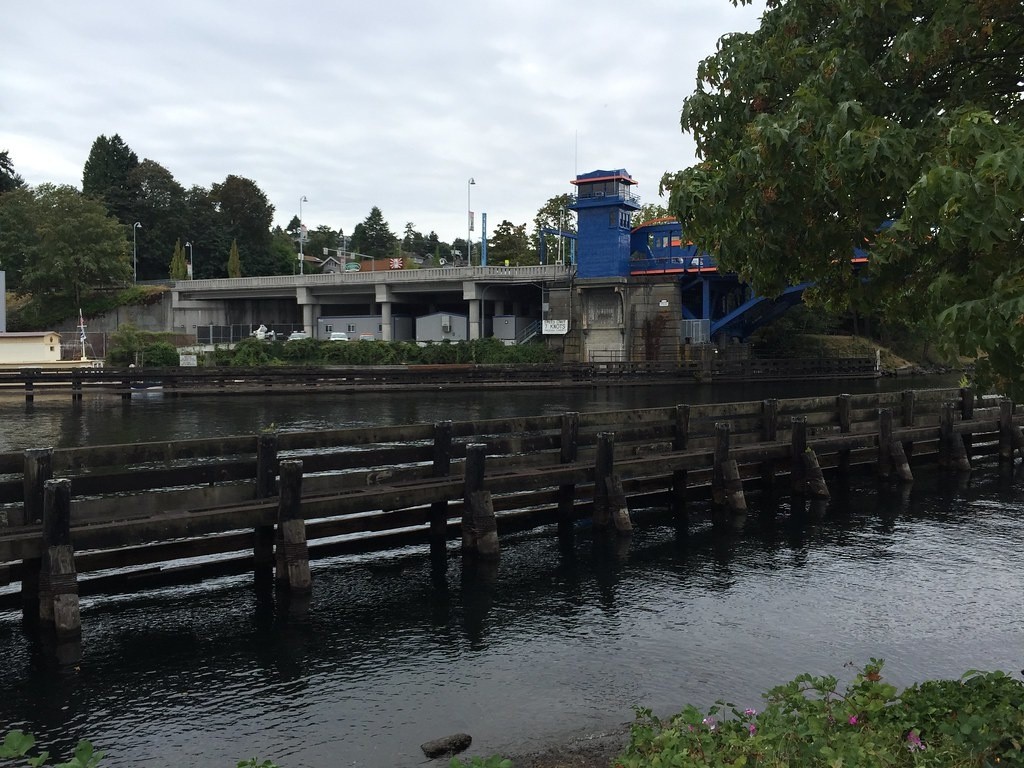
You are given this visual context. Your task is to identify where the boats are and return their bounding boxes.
[0,309,105,384]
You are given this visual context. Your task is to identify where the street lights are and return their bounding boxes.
[339,234,347,273]
[132,221,142,285]
[558,204,567,263]
[300,194,309,275]
[467,178,476,267]
[185,242,193,280]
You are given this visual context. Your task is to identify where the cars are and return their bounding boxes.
[287,331,307,341]
[328,332,351,343]
[360,333,377,342]
